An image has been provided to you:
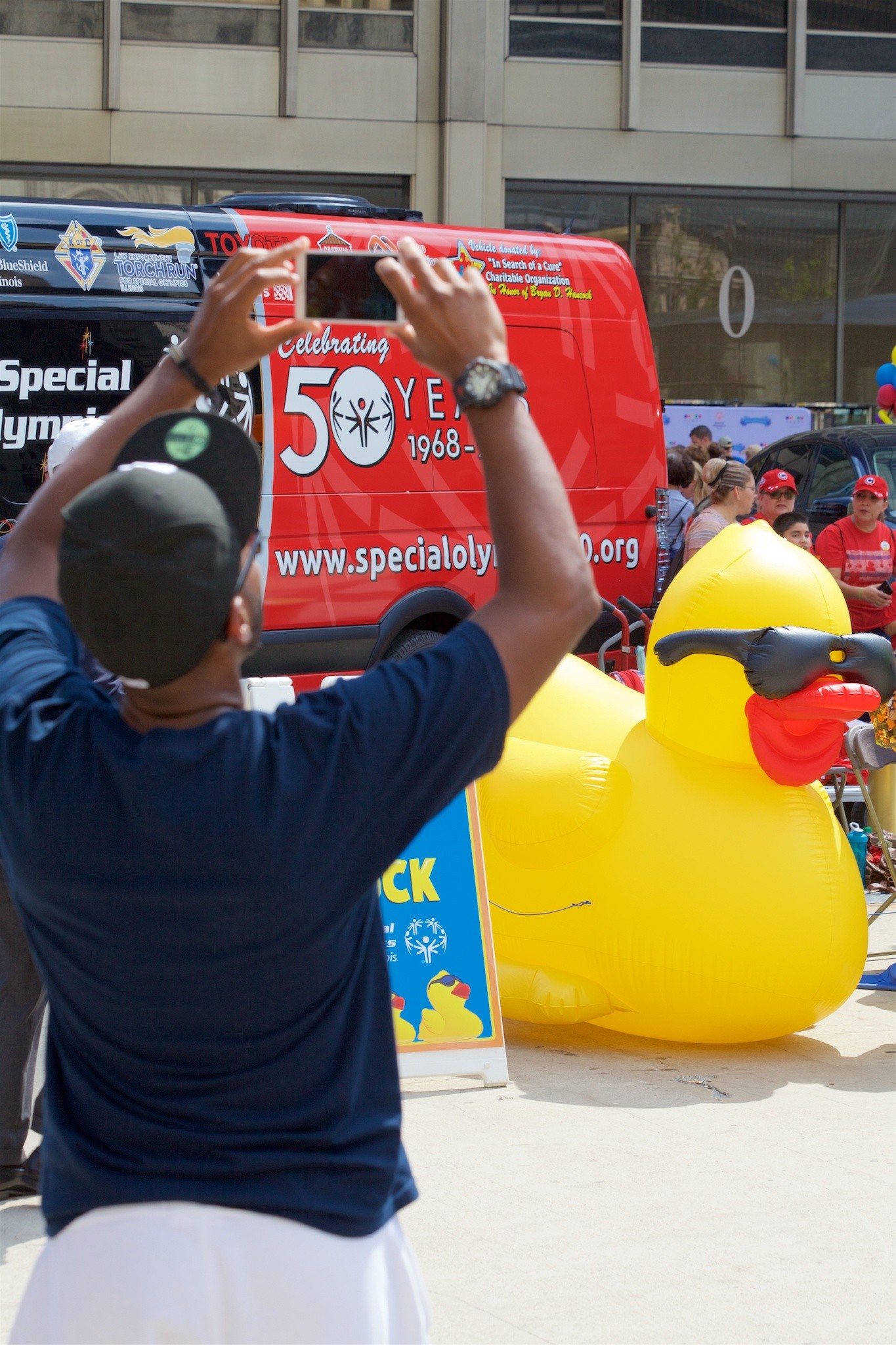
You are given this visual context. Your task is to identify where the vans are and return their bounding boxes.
[0,191,667,687]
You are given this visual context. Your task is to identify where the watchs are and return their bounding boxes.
[453,357,528,411]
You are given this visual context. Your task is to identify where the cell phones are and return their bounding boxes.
[295,251,410,326]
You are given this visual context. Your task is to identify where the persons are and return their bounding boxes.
[773,512,811,551]
[0,418,111,1204]
[742,469,814,556]
[816,475,896,657]
[667,425,763,565]
[683,458,758,567]
[0,235,603,1345]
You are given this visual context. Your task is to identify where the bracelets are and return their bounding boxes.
[169,343,215,398]
[858,587,863,599]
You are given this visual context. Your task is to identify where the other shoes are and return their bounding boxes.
[0,1151,43,1202]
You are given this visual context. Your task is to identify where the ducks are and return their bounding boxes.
[475,520,869,1044]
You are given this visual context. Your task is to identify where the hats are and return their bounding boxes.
[742,445,762,458]
[55,413,263,682]
[851,475,889,502]
[718,437,733,449]
[758,469,798,496]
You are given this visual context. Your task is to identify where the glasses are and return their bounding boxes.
[762,491,795,500]
[220,527,263,645]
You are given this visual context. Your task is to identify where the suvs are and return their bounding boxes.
[661,426,896,548]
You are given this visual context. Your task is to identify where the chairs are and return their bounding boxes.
[824,722,896,926]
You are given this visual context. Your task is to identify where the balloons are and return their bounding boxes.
[876,345,896,424]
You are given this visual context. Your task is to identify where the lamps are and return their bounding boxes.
[581,223,642,242]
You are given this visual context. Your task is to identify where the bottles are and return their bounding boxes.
[847,822,868,887]
[862,827,872,837]
